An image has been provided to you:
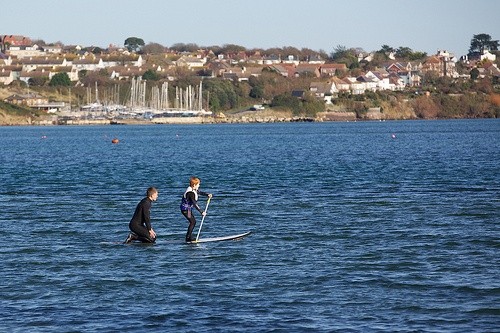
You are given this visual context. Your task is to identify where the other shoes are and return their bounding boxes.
[185,237,196,242]
[124,232,134,244]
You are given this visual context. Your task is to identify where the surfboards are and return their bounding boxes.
[99,231,251,246]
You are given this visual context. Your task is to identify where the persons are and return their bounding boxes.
[180,177,212,242]
[123,186,158,243]
